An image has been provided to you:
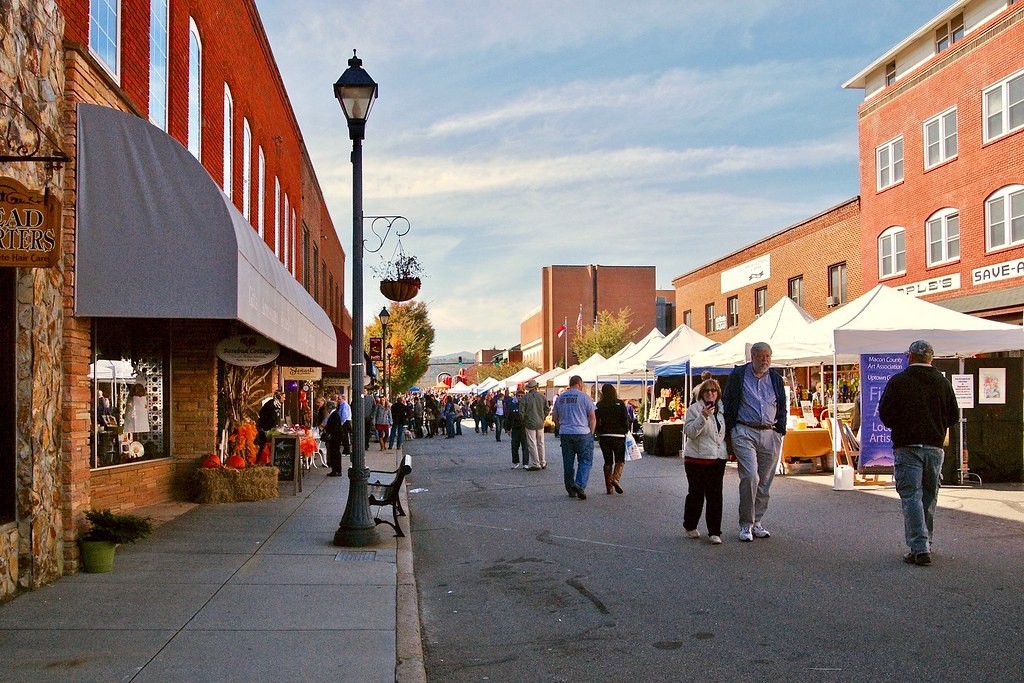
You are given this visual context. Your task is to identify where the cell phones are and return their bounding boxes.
[706,401,713,411]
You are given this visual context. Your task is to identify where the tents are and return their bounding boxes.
[770,284,1024,487]
[409,386,420,394]
[648,297,859,465]
[446,326,666,432]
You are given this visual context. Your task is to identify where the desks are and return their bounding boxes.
[790,405,829,429]
[641,420,683,456]
[782,429,831,474]
[298,435,318,468]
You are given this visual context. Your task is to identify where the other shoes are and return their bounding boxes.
[709,536,721,544]
[511,464,520,469]
[526,467,541,471]
[903,552,931,564]
[523,465,529,469]
[684,528,700,538]
[327,472,342,477]
[543,462,547,468]
[569,484,586,500]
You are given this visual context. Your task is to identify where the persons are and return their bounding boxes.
[724,342,787,541]
[552,375,598,499]
[692,370,724,415]
[406,391,470,439]
[595,384,629,494]
[315,392,352,477]
[470,390,514,442]
[682,379,729,544]
[506,380,549,470]
[879,341,960,564]
[553,387,565,437]
[363,387,407,451]
[257,388,286,440]
[646,386,651,401]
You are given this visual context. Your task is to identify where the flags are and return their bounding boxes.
[556,324,567,338]
[576,309,583,330]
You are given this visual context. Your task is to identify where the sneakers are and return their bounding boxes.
[752,525,770,538]
[739,526,753,541]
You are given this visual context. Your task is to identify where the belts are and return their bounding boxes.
[736,420,774,429]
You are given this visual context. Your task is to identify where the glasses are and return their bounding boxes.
[518,395,524,397]
[704,388,717,392]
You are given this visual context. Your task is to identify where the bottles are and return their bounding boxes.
[300,424,313,438]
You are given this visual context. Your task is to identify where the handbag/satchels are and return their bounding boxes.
[320,432,331,441]
[625,430,643,461]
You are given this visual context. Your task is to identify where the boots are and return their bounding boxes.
[603,464,613,493]
[611,461,623,494]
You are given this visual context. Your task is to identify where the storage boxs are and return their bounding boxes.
[785,461,816,475]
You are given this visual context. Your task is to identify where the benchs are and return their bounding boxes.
[366,451,414,539]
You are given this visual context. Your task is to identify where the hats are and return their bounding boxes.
[526,380,539,390]
[908,339,934,356]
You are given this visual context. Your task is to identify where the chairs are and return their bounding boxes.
[311,435,328,469]
[825,417,886,486]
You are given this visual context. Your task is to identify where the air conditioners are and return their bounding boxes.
[826,296,838,306]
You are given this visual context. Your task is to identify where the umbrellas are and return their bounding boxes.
[433,382,449,388]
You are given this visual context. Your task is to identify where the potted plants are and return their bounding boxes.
[79,507,157,572]
[368,245,430,303]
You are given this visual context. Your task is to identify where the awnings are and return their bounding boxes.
[322,317,366,376]
[364,350,378,376]
[78,99,336,369]
